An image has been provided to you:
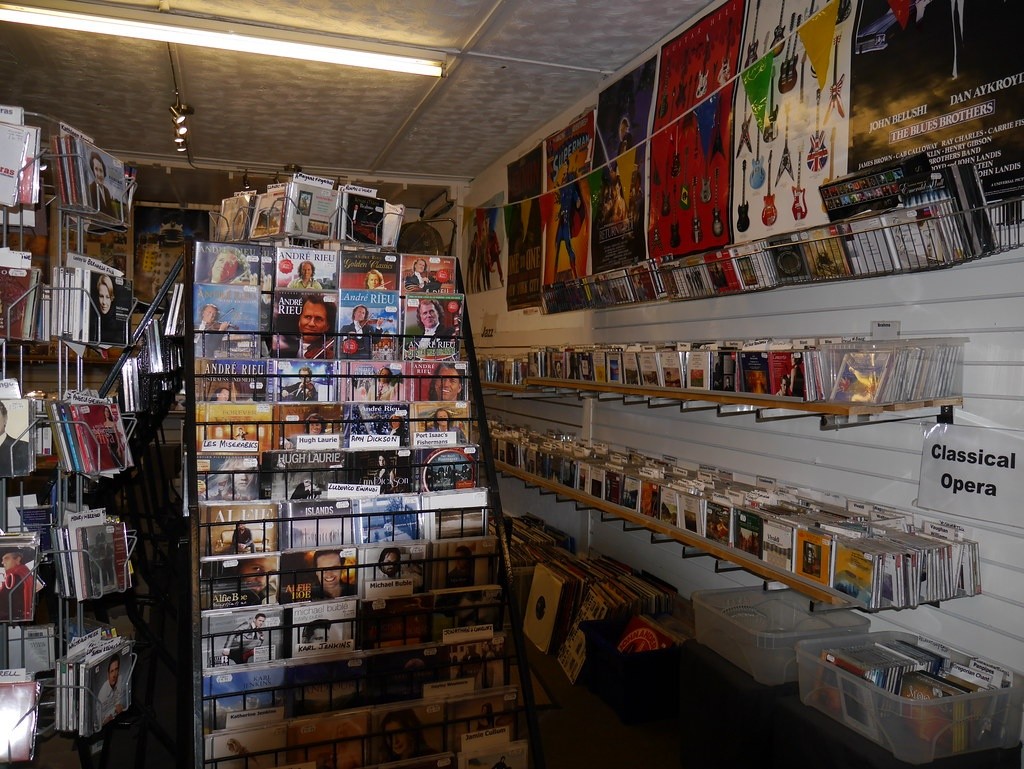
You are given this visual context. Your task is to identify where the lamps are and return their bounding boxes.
[169,93,185,124]
[172,118,188,135]
[177,142,186,153]
[174,127,185,143]
[0,0,448,80]
[243,169,250,189]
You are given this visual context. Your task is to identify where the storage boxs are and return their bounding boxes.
[691,586,871,686]
[794,631,1024,766]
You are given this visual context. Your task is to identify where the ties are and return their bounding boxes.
[253,628,258,640]
[378,385,383,400]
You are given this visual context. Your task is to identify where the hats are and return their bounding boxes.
[0,547,24,559]
